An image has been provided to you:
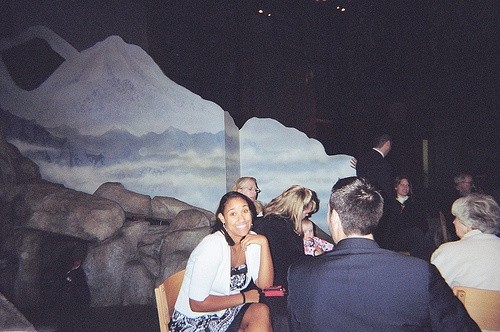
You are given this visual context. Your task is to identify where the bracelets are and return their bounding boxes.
[240,291,246,304]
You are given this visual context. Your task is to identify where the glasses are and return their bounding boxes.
[238,187,260,193]
[399,183,410,188]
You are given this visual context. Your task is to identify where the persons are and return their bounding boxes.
[286,176,483,332]
[231,136,478,264]
[430,193,500,290]
[169,192,274,332]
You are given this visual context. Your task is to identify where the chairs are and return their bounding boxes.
[154,270,185,332]
[453,286,500,332]
